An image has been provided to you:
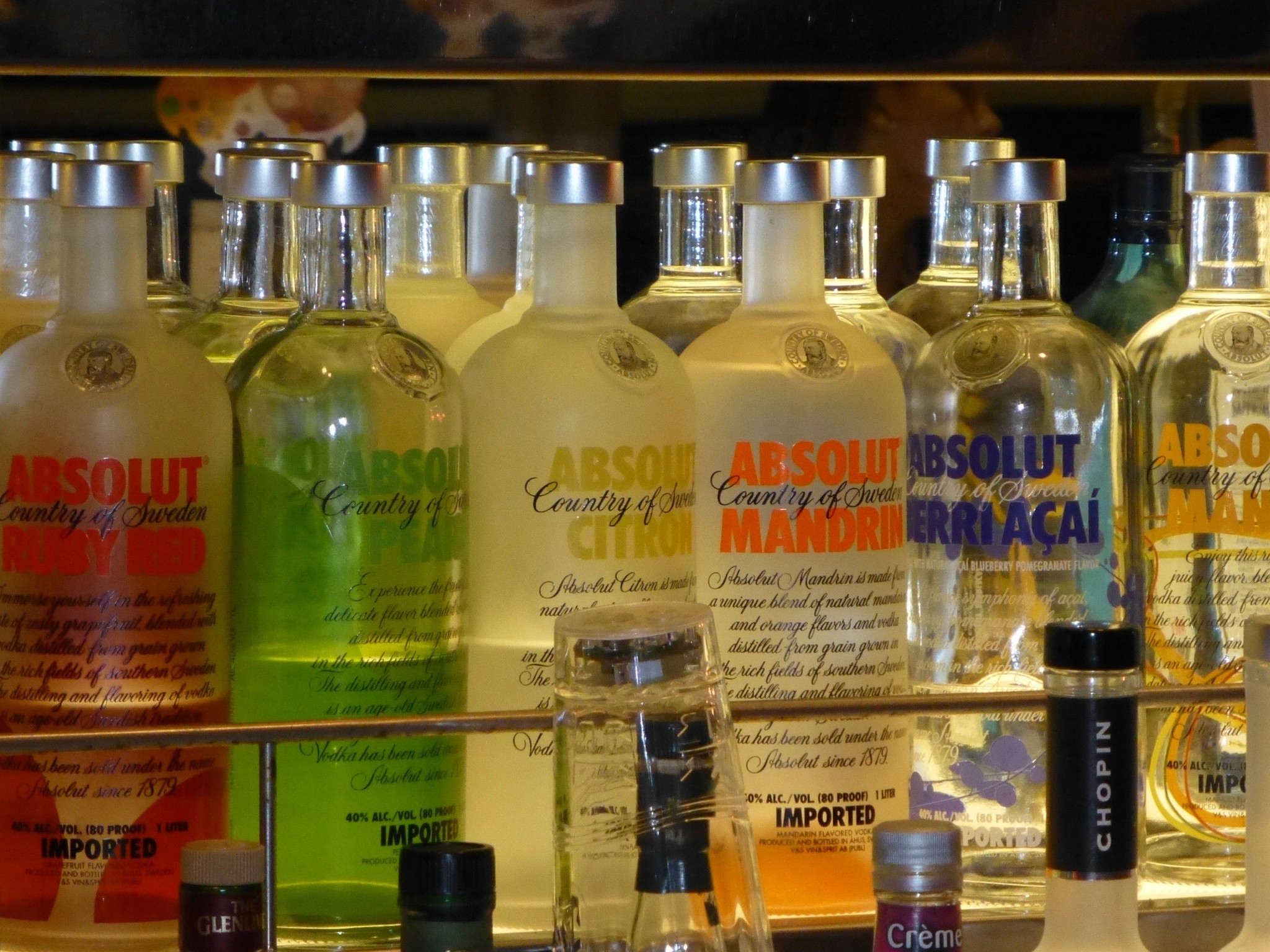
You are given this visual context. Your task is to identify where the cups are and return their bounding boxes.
[547,599,773,952]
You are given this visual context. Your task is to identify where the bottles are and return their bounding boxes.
[821,157,885,297]
[1226,612,1269,952]
[1126,150,1269,951]
[1036,621,1151,952]
[1068,143,1190,347]
[872,820,963,952]
[623,143,749,352]
[679,159,907,933]
[887,137,1019,336]
[905,158,1137,952]
[2,140,725,952]
